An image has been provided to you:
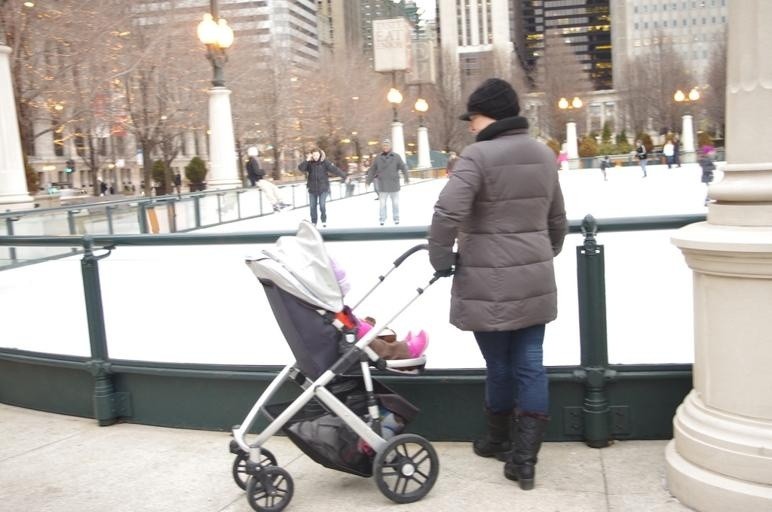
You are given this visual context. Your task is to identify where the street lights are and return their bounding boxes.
[558,95,581,122]
[388,88,403,122]
[197,11,235,87]
[674,88,701,116]
[415,98,428,126]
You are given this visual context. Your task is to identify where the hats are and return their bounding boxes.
[328,257,350,297]
[458,77,520,121]
[703,146,716,155]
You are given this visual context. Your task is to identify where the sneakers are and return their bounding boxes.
[403,328,430,358]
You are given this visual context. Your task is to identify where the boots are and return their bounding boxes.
[473,400,552,490]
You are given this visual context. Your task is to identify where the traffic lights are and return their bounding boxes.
[65,158,74,174]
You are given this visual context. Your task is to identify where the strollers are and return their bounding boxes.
[230,220,455,512]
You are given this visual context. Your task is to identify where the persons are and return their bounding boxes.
[326,257,429,362]
[698,146,716,207]
[670,133,681,168]
[425,77,567,489]
[363,139,412,225]
[246,146,295,216]
[445,151,462,178]
[599,155,608,182]
[81,170,187,203]
[297,149,350,228]
[663,140,674,169]
[629,139,647,179]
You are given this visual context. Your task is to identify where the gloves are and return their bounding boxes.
[433,268,455,277]
